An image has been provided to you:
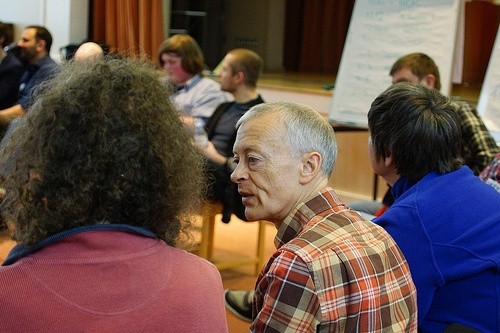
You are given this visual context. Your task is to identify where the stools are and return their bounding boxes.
[183,200,267,280]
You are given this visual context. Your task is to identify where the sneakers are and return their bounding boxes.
[223,288,255,322]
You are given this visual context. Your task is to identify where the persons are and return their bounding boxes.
[230,101,418,333]
[0,48,229,333]
[0,22,500,333]
